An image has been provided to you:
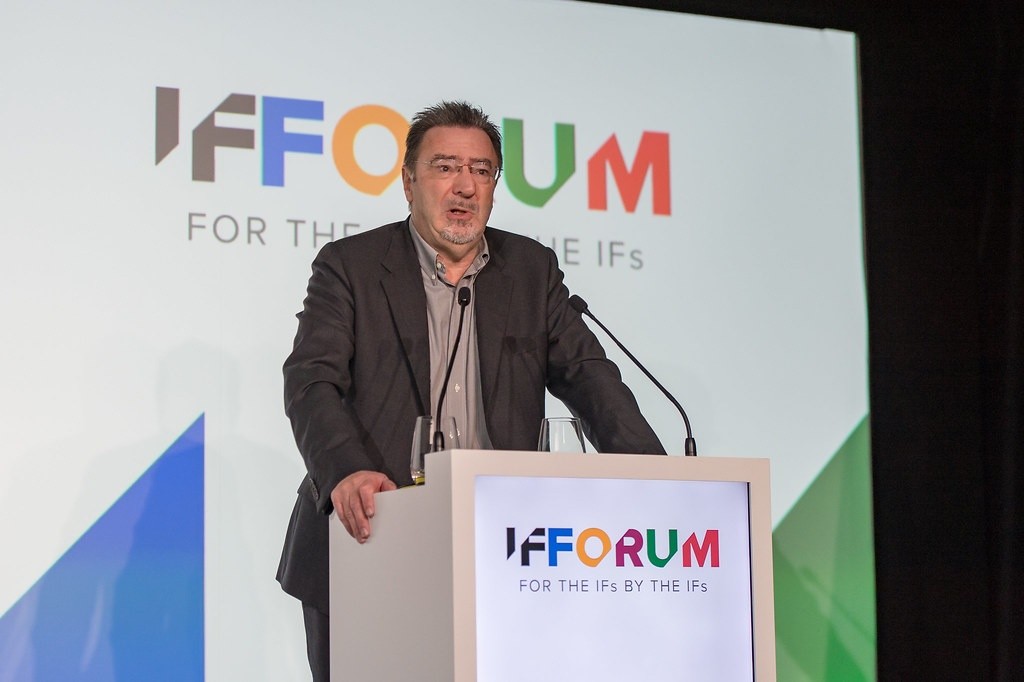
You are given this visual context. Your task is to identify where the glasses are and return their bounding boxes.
[406,158,501,184]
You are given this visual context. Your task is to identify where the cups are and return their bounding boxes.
[409,415,461,485]
[539,417,587,455]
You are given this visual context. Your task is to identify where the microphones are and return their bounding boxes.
[432,287,472,452]
[569,295,696,456]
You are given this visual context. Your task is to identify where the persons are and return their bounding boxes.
[274,102,667,682]
